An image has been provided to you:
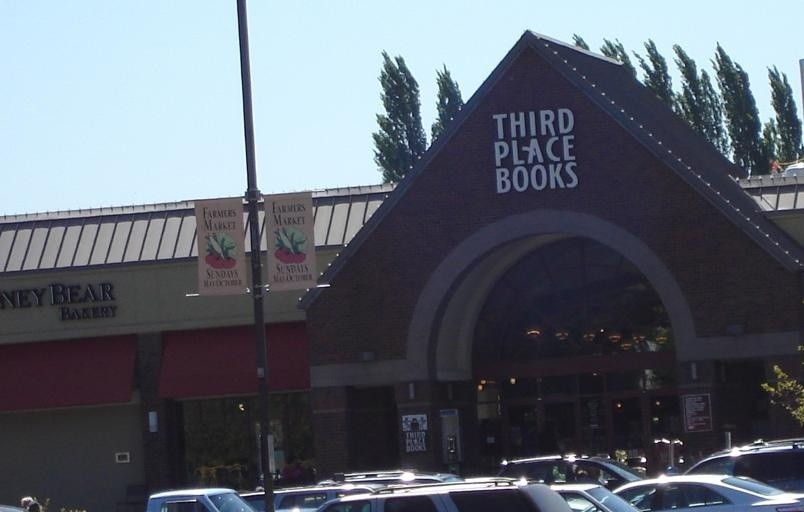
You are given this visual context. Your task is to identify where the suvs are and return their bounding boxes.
[685,436,804,496]
[316,476,573,512]
[497,456,638,487]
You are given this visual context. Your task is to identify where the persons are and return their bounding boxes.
[283,455,305,481]
[583,465,602,485]
[21,497,31,512]
[29,503,40,512]
[300,448,315,481]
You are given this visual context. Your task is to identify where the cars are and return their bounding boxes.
[546,481,641,512]
[582,474,804,512]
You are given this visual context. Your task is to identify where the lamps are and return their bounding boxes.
[447,434,457,465]
[148,411,159,433]
